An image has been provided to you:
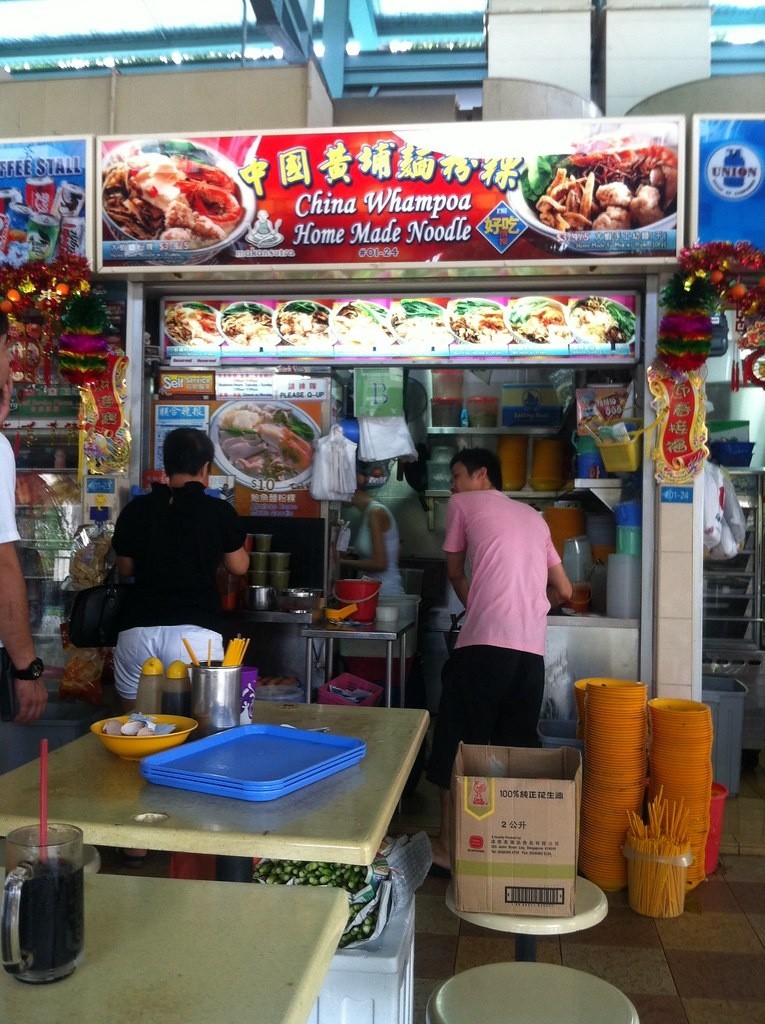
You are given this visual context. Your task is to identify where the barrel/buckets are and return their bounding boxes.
[332,579,382,625]
[705,783,729,875]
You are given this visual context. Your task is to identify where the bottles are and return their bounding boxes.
[163,660,192,716]
[135,657,165,715]
[423,367,498,491]
[55,449,65,468]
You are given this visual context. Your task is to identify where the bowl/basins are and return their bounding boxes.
[90,715,198,762]
[506,140,676,257]
[376,605,397,623]
[218,533,322,614]
[497,435,560,492]
[163,296,638,348]
[541,507,612,613]
[99,139,255,265]
[574,676,714,893]
[210,395,321,494]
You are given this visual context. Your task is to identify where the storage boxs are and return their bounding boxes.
[318,673,379,709]
[449,743,582,918]
[706,419,750,440]
[308,891,417,1023]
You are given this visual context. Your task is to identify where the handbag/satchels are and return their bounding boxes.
[66,563,140,648]
[702,460,746,560]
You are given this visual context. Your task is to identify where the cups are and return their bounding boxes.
[613,500,642,556]
[1,824,83,986]
[571,430,608,479]
[190,660,242,737]
[239,667,258,725]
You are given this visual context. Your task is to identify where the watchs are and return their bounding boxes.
[12,657,44,680]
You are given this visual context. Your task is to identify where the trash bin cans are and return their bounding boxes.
[701,677,749,796]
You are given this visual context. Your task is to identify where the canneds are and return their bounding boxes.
[0,176,85,263]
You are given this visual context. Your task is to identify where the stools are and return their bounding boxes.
[425,963,639,1024]
[446,874,607,960]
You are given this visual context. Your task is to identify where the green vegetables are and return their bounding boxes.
[283,302,329,314]
[222,303,262,316]
[520,155,569,201]
[454,300,500,315]
[220,420,315,441]
[184,303,213,313]
[351,302,386,324]
[401,300,441,317]
[509,301,547,324]
[161,140,216,167]
[606,303,636,337]
[255,860,378,948]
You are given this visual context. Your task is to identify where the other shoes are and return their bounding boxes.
[114,846,149,869]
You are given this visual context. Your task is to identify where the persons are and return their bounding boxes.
[112,427,250,717]
[0,306,51,777]
[338,490,418,707]
[423,446,573,880]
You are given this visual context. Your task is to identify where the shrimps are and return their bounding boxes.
[174,153,243,224]
[276,428,312,470]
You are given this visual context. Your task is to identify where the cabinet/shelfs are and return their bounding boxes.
[2,401,87,641]
[419,367,587,502]
[703,468,764,650]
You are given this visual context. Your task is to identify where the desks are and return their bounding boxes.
[0,697,428,886]
[298,619,418,706]
[0,871,352,1024]
